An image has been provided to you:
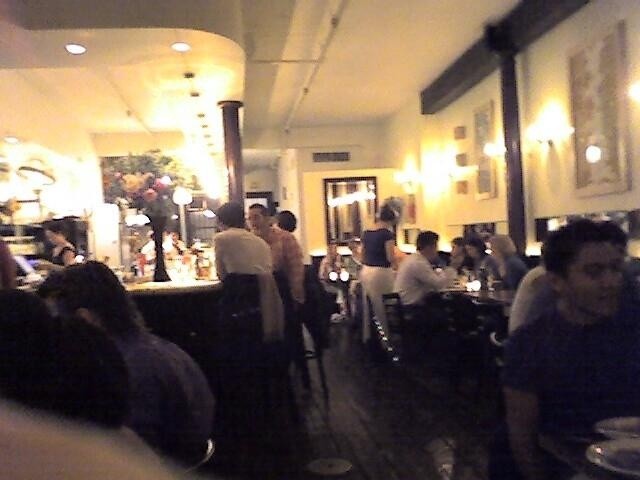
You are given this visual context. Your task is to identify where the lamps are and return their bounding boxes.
[16,154,61,220]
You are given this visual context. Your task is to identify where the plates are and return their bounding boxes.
[584,438,640,477]
[592,413,640,439]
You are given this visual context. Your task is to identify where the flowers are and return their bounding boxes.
[102,148,196,219]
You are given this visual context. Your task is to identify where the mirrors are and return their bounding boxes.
[322,177,377,246]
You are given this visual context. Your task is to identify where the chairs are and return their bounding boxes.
[383,294,507,422]
[219,264,331,416]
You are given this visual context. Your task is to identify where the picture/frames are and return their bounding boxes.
[563,19,633,198]
[470,94,496,201]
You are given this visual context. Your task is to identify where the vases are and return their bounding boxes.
[146,213,170,281]
